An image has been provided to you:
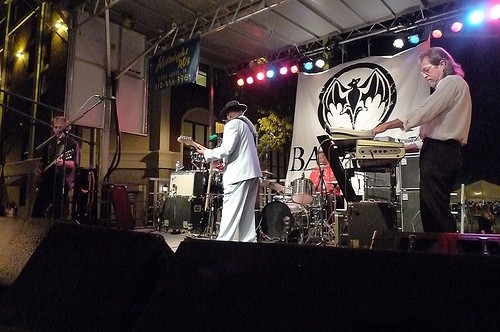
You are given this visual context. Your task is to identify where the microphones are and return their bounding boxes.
[326,131,338,148]
[94,94,116,100]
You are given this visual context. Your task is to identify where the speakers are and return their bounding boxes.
[363,153,420,189]
[169,172,207,197]
[365,186,425,234]
[0,220,175,332]
[153,233,500,332]
[346,197,394,241]
[158,195,209,233]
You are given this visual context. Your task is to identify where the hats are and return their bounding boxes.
[219,101,247,118]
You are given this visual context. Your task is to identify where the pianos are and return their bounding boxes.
[317,128,406,201]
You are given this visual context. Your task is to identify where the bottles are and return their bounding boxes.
[175,159,180,172]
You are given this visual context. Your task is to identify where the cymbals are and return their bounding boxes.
[262,171,276,175]
[326,181,338,184]
[306,167,329,170]
[260,181,284,191]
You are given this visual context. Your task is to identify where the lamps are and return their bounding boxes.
[324,36,338,54]
[236,55,327,88]
[392,17,464,50]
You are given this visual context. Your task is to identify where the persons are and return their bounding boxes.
[371,48,472,233]
[306,152,339,230]
[38,115,79,223]
[196,101,264,243]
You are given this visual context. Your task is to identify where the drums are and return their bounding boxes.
[291,178,316,205]
[273,195,304,213]
[307,192,324,208]
[258,201,311,241]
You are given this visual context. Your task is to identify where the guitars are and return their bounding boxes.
[33,149,74,192]
[177,135,224,170]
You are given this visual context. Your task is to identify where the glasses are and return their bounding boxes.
[421,65,434,77]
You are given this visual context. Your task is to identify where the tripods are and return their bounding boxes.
[303,137,336,245]
